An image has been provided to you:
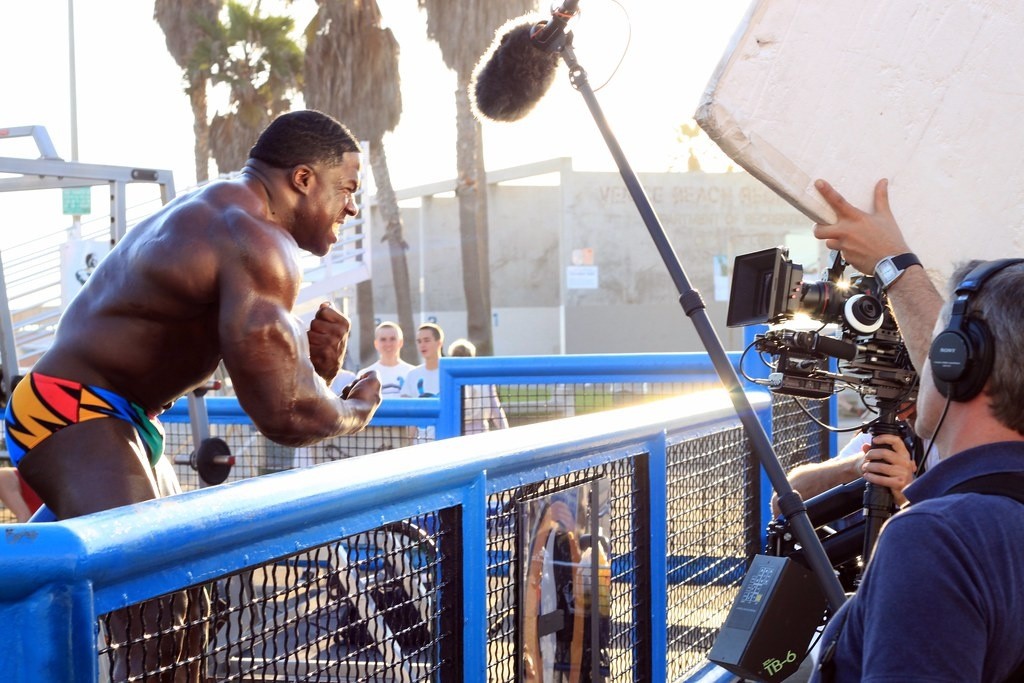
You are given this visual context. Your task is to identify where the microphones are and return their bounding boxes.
[467,0,579,123]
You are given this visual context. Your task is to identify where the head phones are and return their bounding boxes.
[927,258,1024,404]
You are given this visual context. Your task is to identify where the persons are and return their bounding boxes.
[293,321,511,467]
[0,466,43,525]
[769,179,945,521]
[807,258,1024,683]
[4,112,381,683]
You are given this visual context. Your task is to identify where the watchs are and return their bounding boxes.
[873,254,923,290]
